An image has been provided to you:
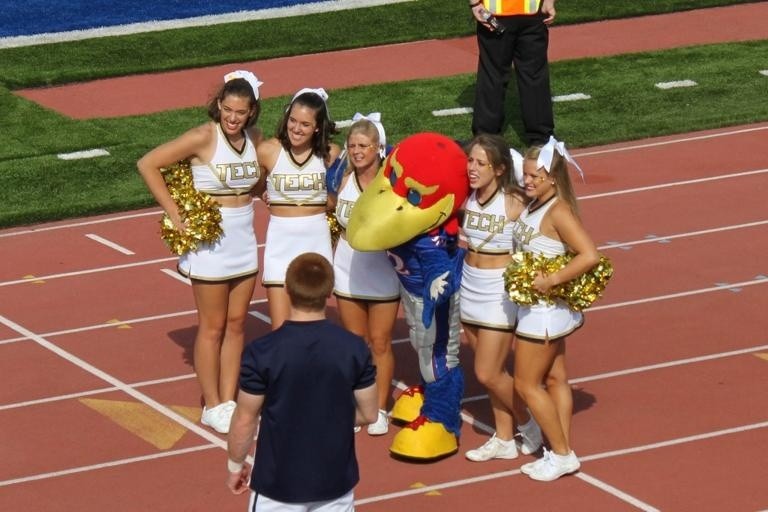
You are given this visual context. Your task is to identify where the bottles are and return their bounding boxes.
[478,7,506,39]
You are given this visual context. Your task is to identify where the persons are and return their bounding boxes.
[469,0,557,147]
[137,70,270,440]
[332,112,401,435]
[257,87,341,330]
[226,251,377,512]
[513,135,601,481]
[459,134,541,462]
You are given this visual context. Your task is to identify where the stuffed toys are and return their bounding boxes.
[326,133,473,459]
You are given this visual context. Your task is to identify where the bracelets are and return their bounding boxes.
[469,1,481,7]
[227,460,243,473]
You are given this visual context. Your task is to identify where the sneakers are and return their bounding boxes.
[464,409,582,481]
[367,409,389,436]
[223,400,235,415]
[201,404,230,434]
